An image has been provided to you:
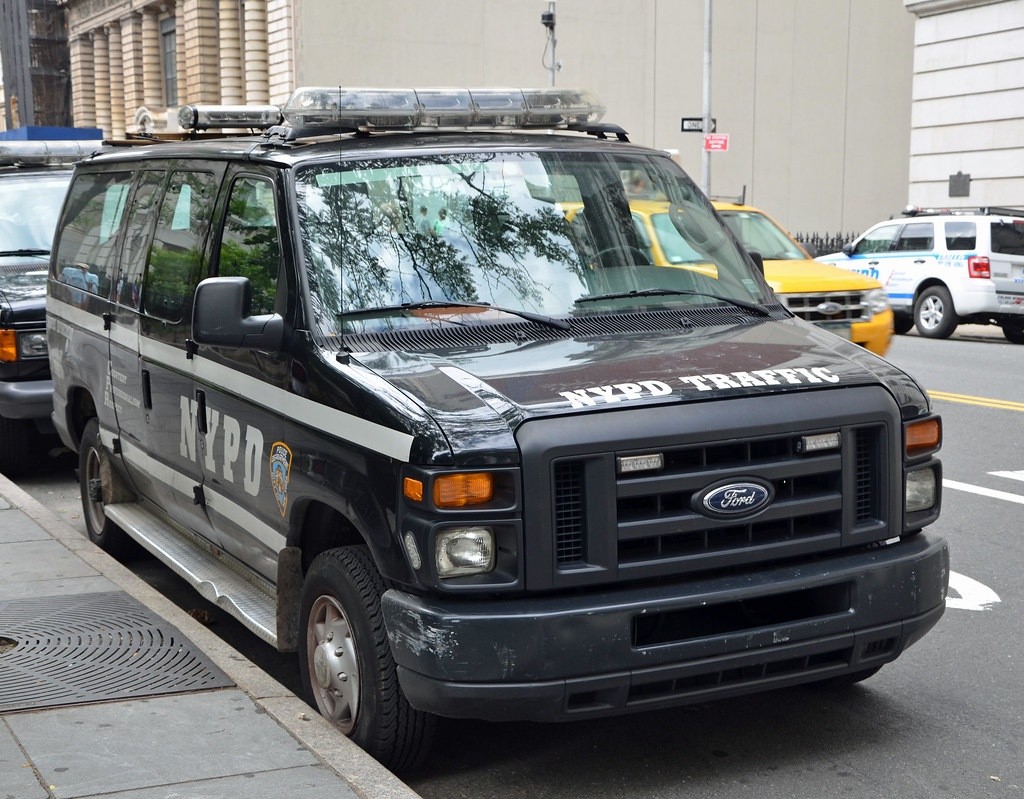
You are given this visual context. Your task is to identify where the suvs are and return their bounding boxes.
[554,194,895,359]
[0,123,118,469]
[812,205,1024,345]
[49,85,944,768]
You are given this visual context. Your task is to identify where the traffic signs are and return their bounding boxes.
[682,117,717,132]
